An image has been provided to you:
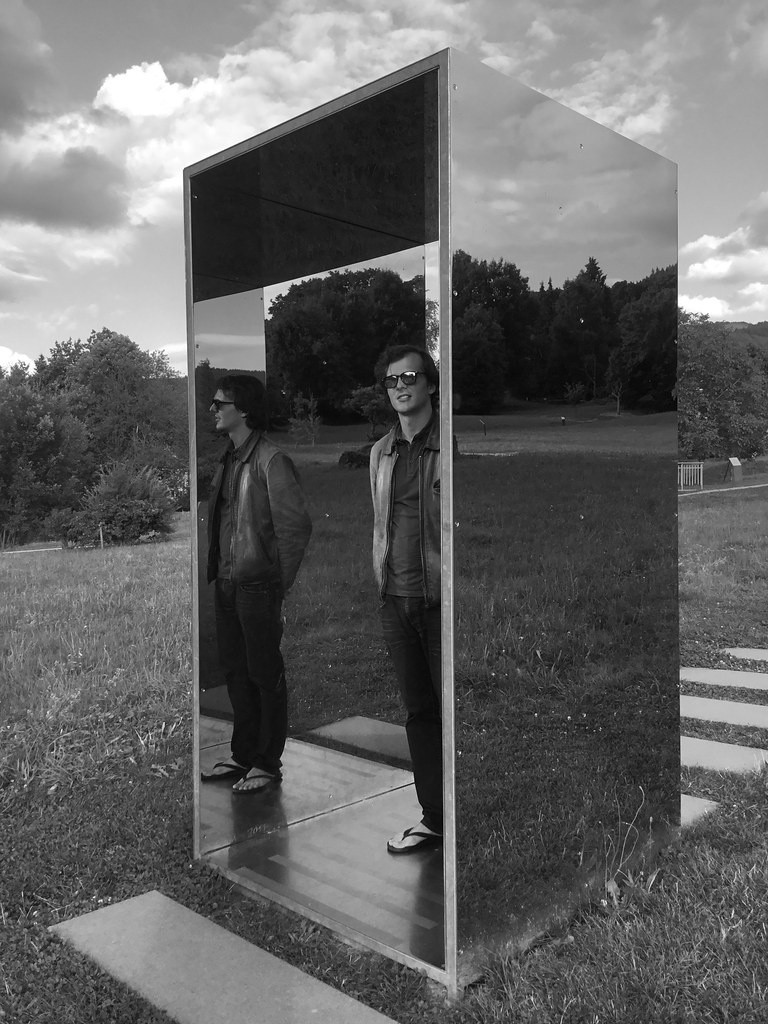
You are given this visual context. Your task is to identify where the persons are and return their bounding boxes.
[370,346,441,855]
[201,374,313,793]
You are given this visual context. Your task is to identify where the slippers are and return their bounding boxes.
[200,760,249,780]
[232,768,283,793]
[387,827,443,853]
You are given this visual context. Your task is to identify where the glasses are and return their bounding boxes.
[382,370,427,388]
[210,398,238,411]
